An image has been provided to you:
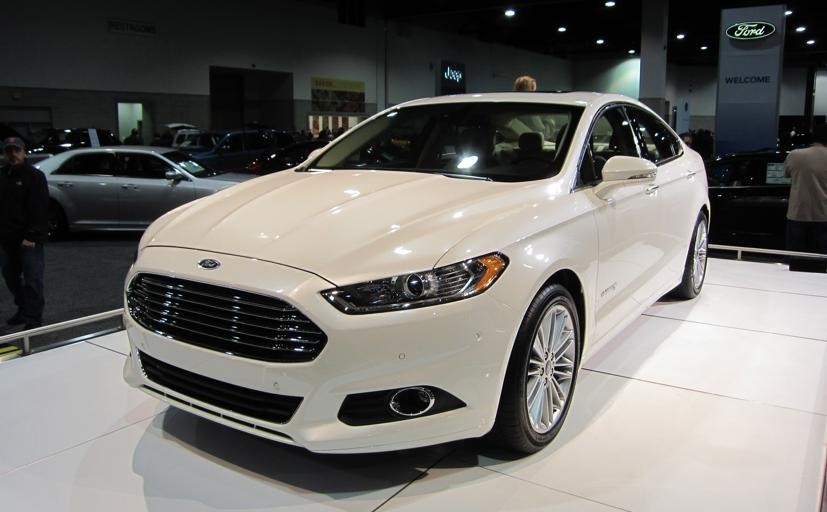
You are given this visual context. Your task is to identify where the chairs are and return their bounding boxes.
[556,124,607,173]
[513,131,554,159]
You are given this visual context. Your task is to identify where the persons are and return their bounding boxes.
[511,76,557,142]
[0,137,63,341]
[782,121,826,273]
[123,127,142,145]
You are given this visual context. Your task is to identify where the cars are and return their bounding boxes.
[705,148,791,250]
[25,145,255,244]
